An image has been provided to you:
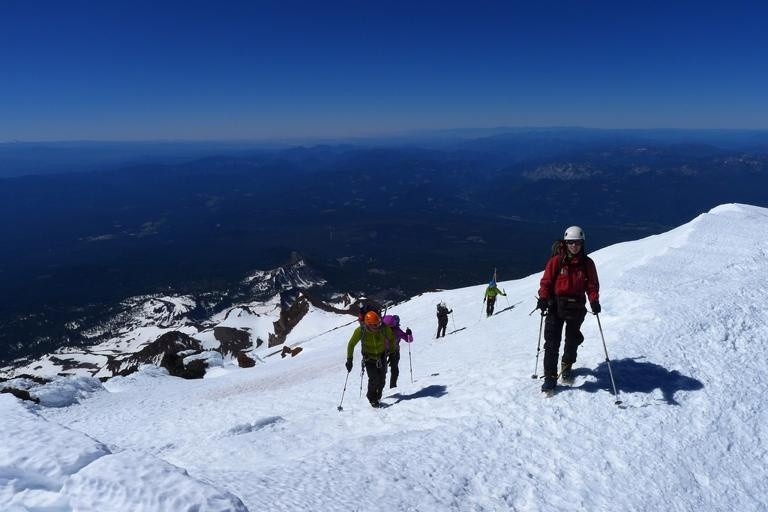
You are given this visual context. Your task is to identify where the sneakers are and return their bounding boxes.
[366,393,380,407]
[540,375,558,391]
[560,357,572,378]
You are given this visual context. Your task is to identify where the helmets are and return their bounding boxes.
[364,310,382,326]
[563,225,586,241]
[441,302,446,308]
[489,281,494,287]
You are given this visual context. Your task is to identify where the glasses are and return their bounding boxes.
[567,240,583,245]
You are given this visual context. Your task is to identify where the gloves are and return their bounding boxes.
[536,298,548,309]
[344,360,353,372]
[590,301,601,315]
[406,329,412,335]
[387,356,395,366]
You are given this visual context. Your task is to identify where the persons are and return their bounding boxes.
[534,225,602,392]
[481,280,506,319]
[380,314,413,389]
[344,311,396,408]
[435,301,452,339]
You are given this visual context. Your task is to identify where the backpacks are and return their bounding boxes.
[357,301,385,327]
[550,240,565,259]
